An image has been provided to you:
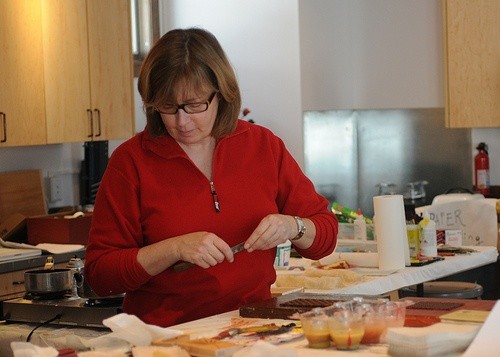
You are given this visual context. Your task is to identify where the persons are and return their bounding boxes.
[83,27,339,328]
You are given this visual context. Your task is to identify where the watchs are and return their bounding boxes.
[291,215,307,241]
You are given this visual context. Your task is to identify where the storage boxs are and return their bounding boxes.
[26,211,92,247]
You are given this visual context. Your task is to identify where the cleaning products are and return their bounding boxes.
[417,206,438,260]
[352,208,367,241]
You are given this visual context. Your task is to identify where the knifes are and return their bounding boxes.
[172,241,247,272]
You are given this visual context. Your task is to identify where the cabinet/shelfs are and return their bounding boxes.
[0,0,47,147]
[443,0,500,129]
[42,0,136,144]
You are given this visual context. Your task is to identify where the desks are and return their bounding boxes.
[271,246,498,301]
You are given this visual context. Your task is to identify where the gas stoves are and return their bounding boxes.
[4,293,128,326]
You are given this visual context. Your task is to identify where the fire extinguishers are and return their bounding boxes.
[474,142,491,195]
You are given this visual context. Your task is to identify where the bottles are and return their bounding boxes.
[419,217,437,258]
[354,209,367,241]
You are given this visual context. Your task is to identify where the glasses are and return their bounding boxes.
[153,90,216,114]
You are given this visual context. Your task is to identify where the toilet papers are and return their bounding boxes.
[372,194,411,271]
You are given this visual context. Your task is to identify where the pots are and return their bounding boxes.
[24,268,84,297]
